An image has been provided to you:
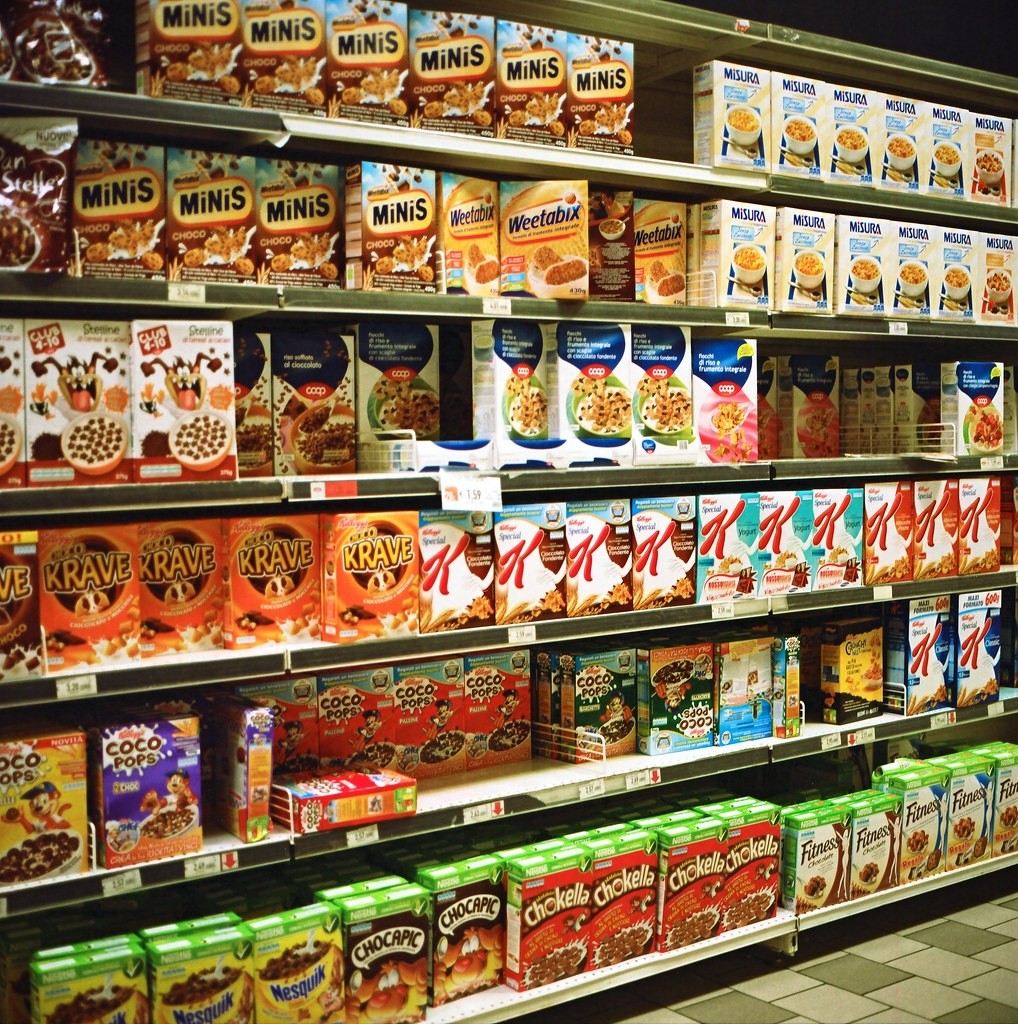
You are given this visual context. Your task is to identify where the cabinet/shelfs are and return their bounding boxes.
[0,0,1018,1024]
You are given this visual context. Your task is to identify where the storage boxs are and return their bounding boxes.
[0,0,1018,1024]
